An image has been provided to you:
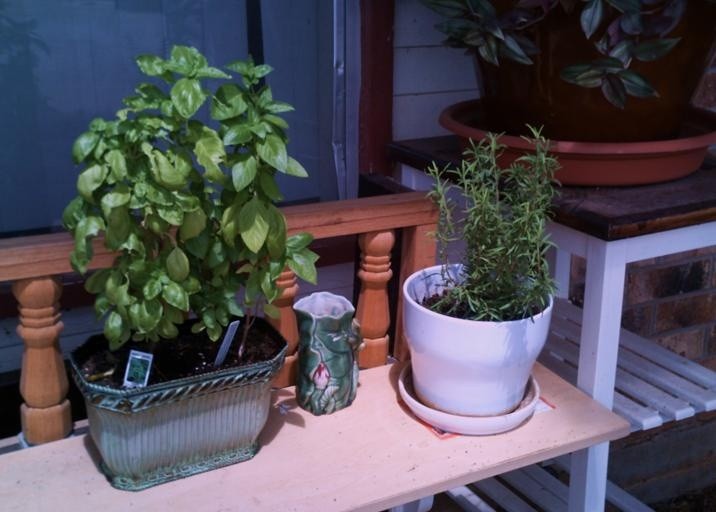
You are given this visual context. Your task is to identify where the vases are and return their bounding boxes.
[293,289,358,415]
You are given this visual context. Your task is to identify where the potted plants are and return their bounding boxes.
[394,125,563,436]
[422,1,715,185]
[67,48,318,493]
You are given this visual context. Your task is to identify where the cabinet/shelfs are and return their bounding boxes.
[1,194,626,511]
[387,134,715,511]
